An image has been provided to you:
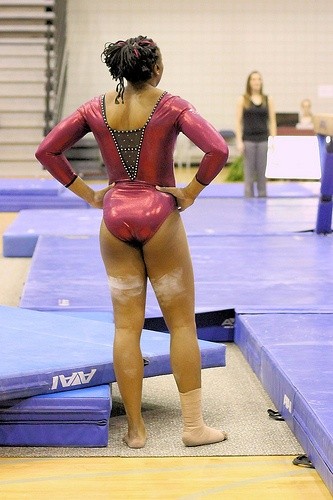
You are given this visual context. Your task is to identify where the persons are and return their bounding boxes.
[236,70,277,198]
[299,98,313,125]
[35,34,229,450]
